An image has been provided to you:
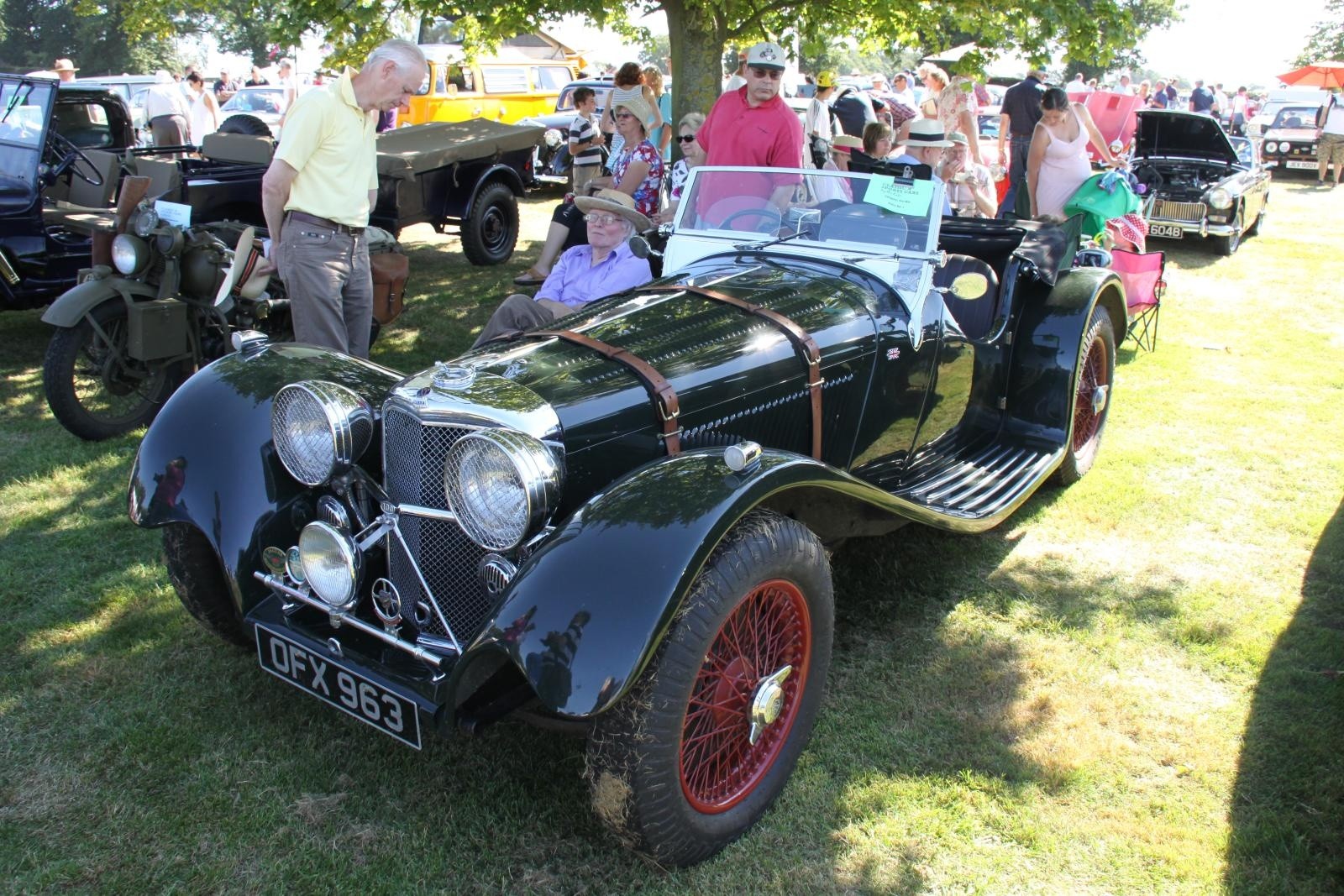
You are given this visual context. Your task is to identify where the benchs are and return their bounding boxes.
[801,200,1027,347]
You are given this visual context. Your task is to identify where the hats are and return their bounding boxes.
[154,70,174,83]
[817,70,840,87]
[946,132,968,145]
[738,53,748,61]
[835,86,858,98]
[829,135,864,155]
[747,43,785,70]
[50,59,80,71]
[213,226,272,307]
[573,188,650,233]
[897,119,955,147]
[871,74,884,81]
[1106,214,1148,253]
[613,98,651,137]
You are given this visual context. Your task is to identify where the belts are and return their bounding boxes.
[291,212,365,237]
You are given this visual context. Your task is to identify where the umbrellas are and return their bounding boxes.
[1276,60,1344,94]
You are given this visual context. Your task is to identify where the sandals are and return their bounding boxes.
[514,268,550,286]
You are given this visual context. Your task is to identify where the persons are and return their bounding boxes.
[652,112,706,224]
[998,66,1049,220]
[569,52,672,189]
[802,53,998,218]
[681,42,805,232]
[1066,73,1267,136]
[376,108,398,131]
[259,39,427,359]
[1026,87,1130,220]
[1086,214,1149,253]
[473,189,652,346]
[726,48,751,91]
[1286,112,1301,127]
[1315,85,1344,188]
[52,59,100,123]
[514,97,663,283]
[142,59,299,159]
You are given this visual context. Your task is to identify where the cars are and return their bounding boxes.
[1,29,670,444]
[126,172,1130,872]
[782,74,1344,256]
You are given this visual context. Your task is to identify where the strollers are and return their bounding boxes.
[1056,171,1143,250]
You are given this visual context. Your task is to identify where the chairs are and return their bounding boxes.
[42,149,117,226]
[1107,249,1166,353]
[819,204,908,249]
[60,158,181,237]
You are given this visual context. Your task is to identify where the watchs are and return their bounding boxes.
[590,140,593,147]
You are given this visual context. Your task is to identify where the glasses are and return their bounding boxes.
[751,67,781,80]
[676,134,696,143]
[894,80,900,82]
[616,114,632,118]
[585,213,625,224]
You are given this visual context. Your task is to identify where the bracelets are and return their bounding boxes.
[998,148,1004,151]
[657,148,661,152]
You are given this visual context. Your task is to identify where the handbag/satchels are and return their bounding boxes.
[1315,94,1335,125]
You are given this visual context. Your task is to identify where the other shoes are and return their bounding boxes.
[1317,180,1324,185]
[1332,182,1338,189]
